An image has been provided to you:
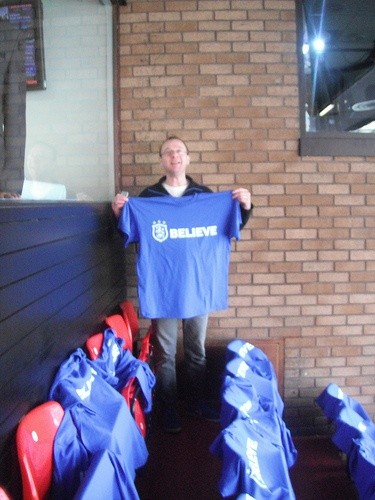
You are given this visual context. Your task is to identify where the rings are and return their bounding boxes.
[114,202,117,207]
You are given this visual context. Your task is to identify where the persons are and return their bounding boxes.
[111,136,255,433]
[0,19,27,201]
[26,141,55,181]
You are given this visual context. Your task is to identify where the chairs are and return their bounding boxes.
[209,336,375,500]
[0,300,159,500]
